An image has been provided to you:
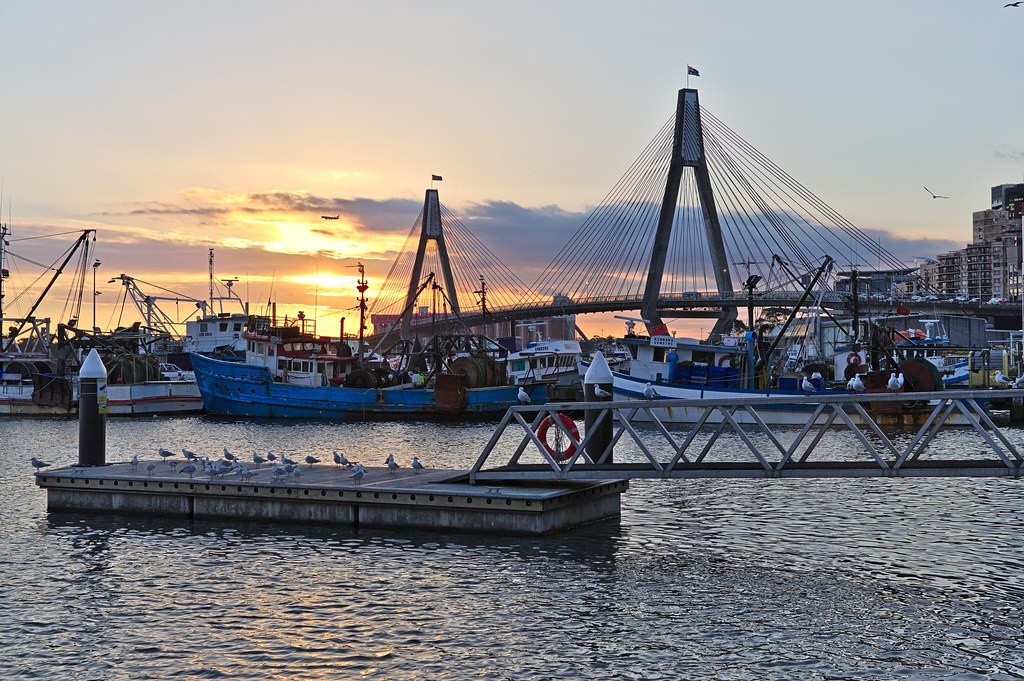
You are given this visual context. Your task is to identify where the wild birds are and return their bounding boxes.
[412,456,425,475]
[593,383,612,402]
[885,372,905,396]
[130,448,177,476]
[333,451,359,470]
[802,376,818,396]
[167,460,181,472]
[847,374,866,396]
[643,382,660,401]
[518,387,531,405]
[95,258,100,262]
[305,456,321,468]
[383,454,399,474]
[252,450,268,469]
[992,370,1014,390]
[923,186,949,199]
[182,449,204,463]
[179,464,197,478]
[1004,1,1024,8]
[202,448,257,483]
[348,464,368,485]
[28,457,51,473]
[266,451,304,484]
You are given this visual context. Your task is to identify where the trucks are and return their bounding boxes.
[553,296,573,304]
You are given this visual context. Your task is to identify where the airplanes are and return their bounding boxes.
[321,215,339,220]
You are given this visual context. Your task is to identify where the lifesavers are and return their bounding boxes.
[718,355,735,368]
[847,353,861,365]
[537,414,580,461]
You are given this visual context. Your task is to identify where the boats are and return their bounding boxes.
[0,188,371,416]
[189,262,584,414]
[581,233,986,426]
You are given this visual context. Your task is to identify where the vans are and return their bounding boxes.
[683,292,701,300]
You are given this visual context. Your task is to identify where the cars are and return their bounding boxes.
[859,293,885,299]
[911,295,1006,304]
